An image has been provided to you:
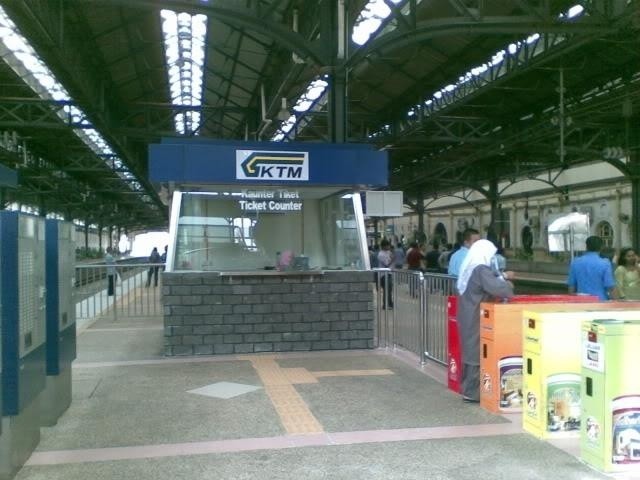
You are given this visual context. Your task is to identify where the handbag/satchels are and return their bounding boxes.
[114,272,123,288]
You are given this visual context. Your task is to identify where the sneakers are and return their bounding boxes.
[462,396,482,404]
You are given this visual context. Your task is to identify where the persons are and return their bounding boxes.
[567,235,617,301]
[613,246,640,301]
[105,247,118,296]
[369,227,516,403]
[145,247,160,286]
[161,245,167,271]
[277,249,293,269]
